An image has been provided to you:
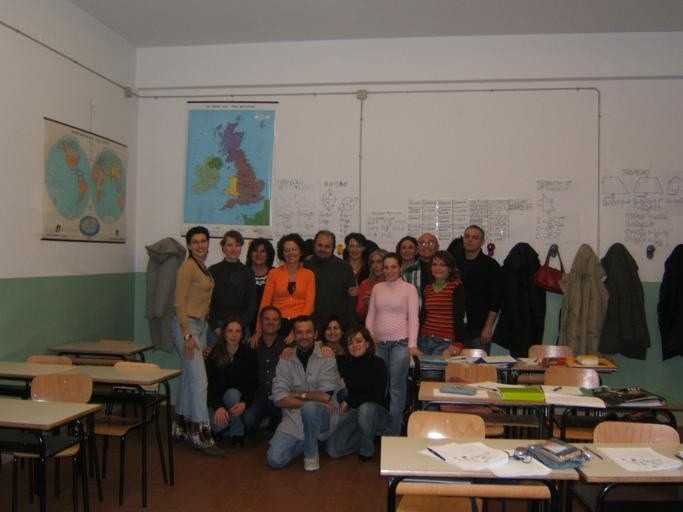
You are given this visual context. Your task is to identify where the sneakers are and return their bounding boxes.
[303,450,320,471]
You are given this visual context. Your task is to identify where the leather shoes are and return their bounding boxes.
[358,454,371,466]
[195,434,248,460]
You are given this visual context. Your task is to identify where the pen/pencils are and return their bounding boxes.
[452,357,467,360]
[584,446,603,460]
[478,386,497,392]
[426,448,446,461]
[534,359,539,362]
[553,387,561,391]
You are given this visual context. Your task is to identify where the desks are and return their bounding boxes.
[0,398,102,512]
[0,358,78,498]
[1,360,184,510]
[54,341,159,360]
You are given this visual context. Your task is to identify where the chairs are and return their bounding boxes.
[413,344,682,435]
[380,411,683,512]
[83,362,168,507]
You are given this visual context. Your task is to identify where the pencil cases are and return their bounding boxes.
[440,384,475,396]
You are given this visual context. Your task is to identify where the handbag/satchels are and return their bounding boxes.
[533,265,569,292]
[568,293,569,294]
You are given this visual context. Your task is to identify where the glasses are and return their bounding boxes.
[282,247,299,253]
[419,241,435,247]
